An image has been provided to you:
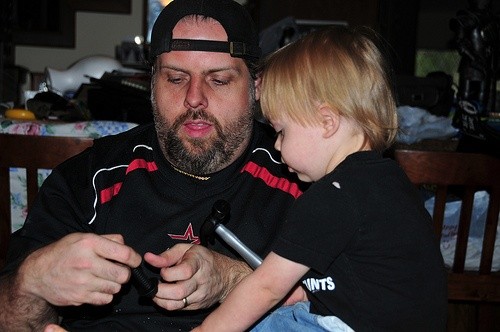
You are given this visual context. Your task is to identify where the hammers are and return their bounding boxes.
[198,201,263,271]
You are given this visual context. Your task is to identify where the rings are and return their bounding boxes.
[182,297,187,308]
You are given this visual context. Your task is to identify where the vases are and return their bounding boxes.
[46,55,123,96]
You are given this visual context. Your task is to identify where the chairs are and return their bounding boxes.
[0,132,93,250]
[395,149,500,332]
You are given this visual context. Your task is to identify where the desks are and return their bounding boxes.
[0,114,140,234]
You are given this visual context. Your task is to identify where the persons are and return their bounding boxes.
[0,0,316,332]
[44,29,449,332]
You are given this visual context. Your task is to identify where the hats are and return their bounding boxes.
[150,0,261,60]
